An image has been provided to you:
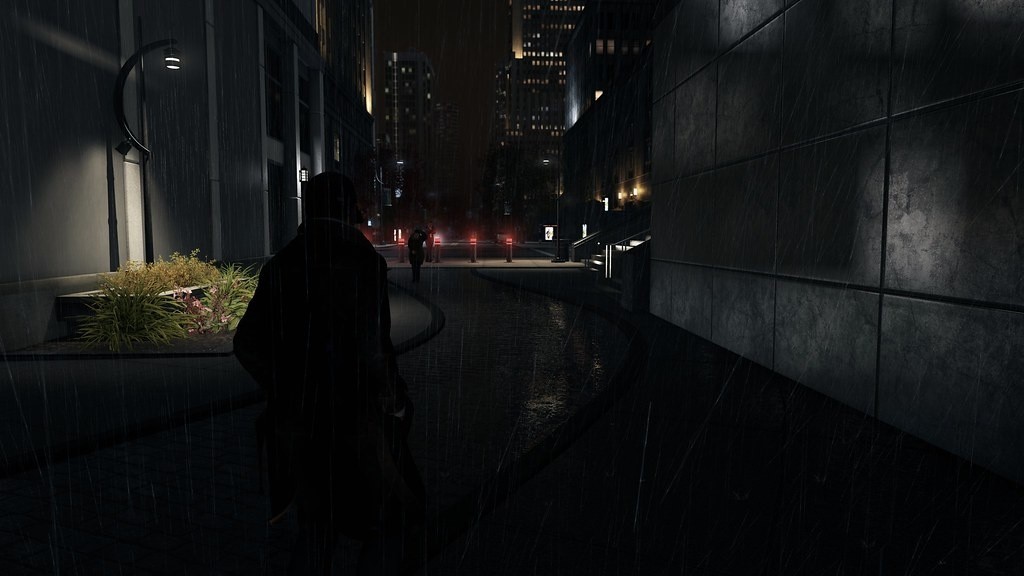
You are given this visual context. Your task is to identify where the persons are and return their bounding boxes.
[232,171,413,576]
[408,230,427,283]
[424,220,436,262]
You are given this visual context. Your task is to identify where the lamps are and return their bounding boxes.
[111,38,182,167]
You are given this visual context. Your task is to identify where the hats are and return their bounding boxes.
[305,171,365,224]
